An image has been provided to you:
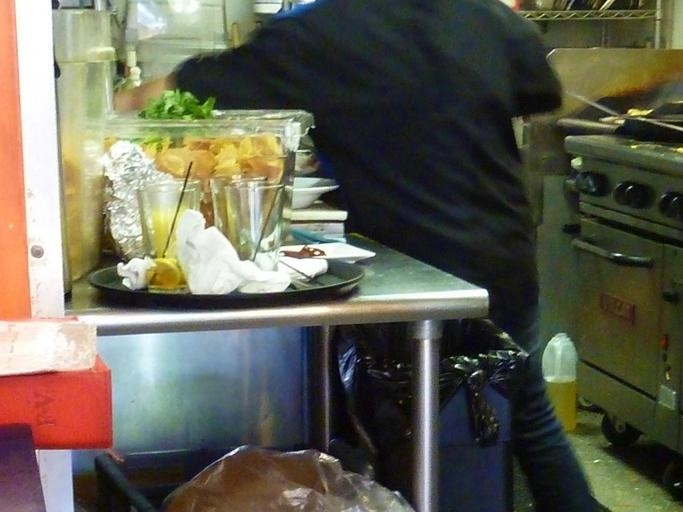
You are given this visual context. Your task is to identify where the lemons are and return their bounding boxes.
[151,257,181,288]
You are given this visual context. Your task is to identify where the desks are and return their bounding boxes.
[62,233,490,511]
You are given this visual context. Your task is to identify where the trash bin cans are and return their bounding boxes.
[337,318,514,512]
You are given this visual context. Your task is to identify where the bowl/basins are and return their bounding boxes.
[284,175,340,209]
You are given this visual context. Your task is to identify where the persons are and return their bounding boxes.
[114,1,610,511]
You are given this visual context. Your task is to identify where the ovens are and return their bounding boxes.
[565,156,682,410]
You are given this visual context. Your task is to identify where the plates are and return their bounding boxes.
[275,238,378,265]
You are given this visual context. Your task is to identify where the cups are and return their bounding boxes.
[225,180,284,271]
[136,179,202,296]
[210,173,268,242]
